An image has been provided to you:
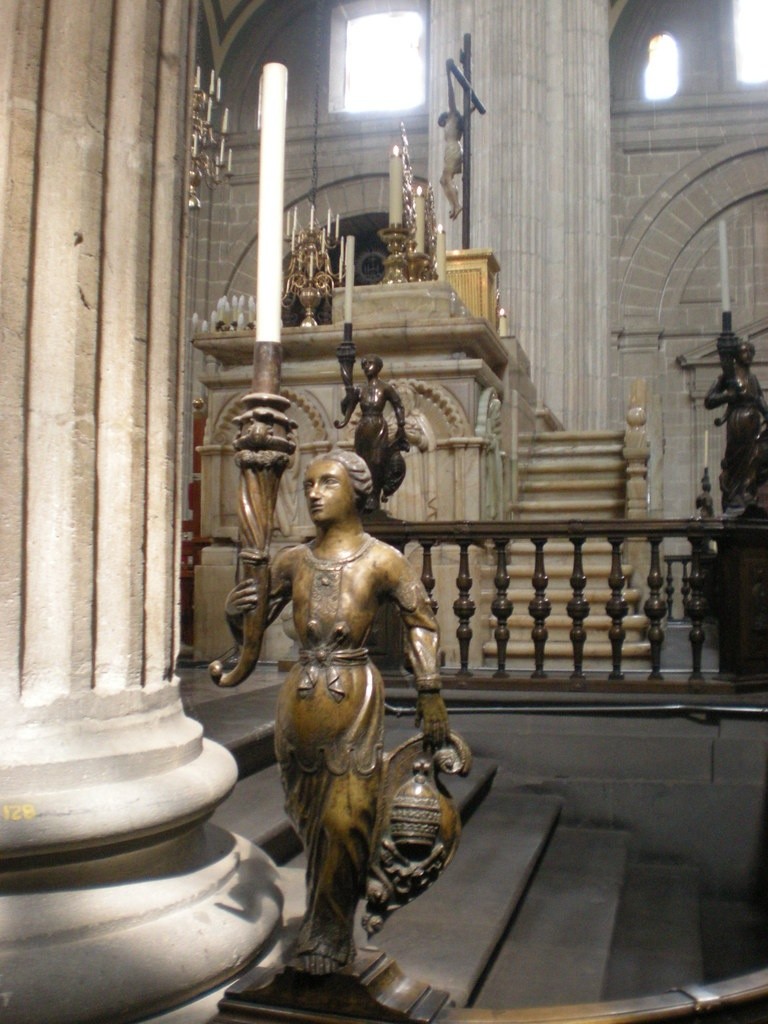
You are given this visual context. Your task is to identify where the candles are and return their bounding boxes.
[388,145,404,228]
[191,65,233,173]
[321,228,326,253]
[286,204,315,251]
[309,252,314,281]
[327,208,331,234]
[415,186,425,253]
[499,308,507,336]
[436,224,447,281]
[338,254,343,283]
[340,236,344,264]
[718,220,728,313]
[705,429,709,467]
[345,235,355,323]
[336,214,340,237]
[256,60,289,343]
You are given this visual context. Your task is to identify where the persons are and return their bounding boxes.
[705,341,768,519]
[340,353,406,513]
[695,481,715,519]
[224,450,450,974]
[438,58,476,220]
